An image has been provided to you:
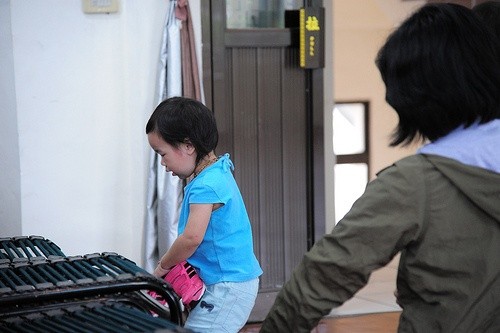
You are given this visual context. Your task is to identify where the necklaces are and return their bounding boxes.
[196,161,208,174]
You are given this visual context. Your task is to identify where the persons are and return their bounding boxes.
[257,1,500,333]
[145,96,263,333]
